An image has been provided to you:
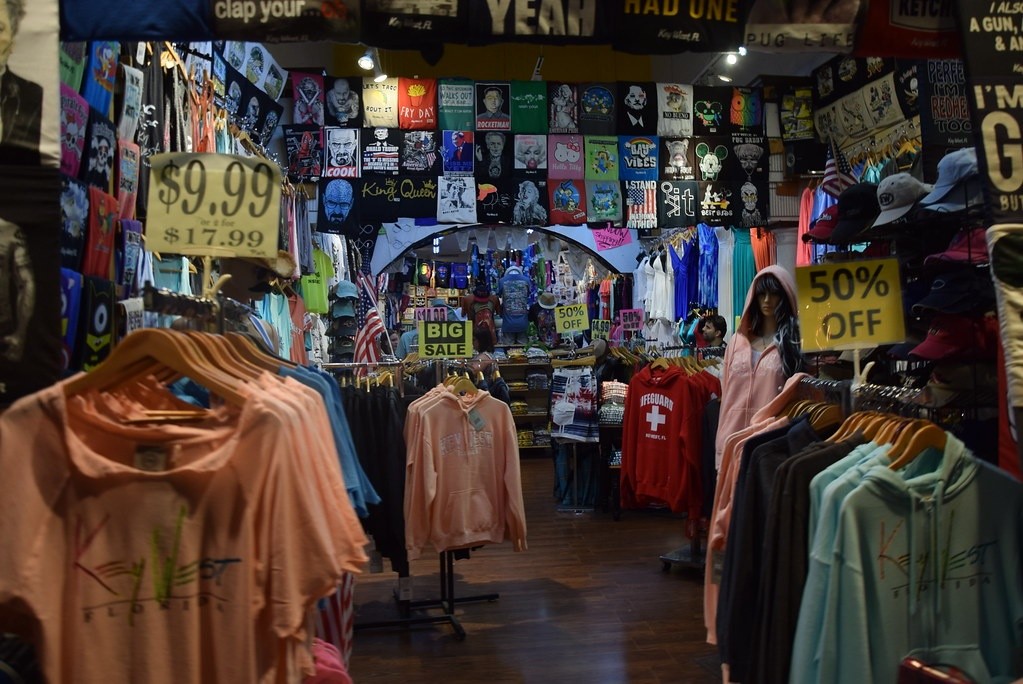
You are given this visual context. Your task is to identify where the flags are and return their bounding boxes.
[822,132,860,199]
[353,278,386,376]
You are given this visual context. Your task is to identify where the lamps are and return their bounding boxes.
[357,47,375,70]
[373,60,388,82]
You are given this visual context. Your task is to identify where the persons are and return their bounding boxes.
[477,87,508,121]
[702,314,728,359]
[441,178,472,208]
[462,285,501,345]
[0,219,36,412]
[551,84,576,128]
[0,1,43,151]
[396,329,419,361]
[621,83,657,134]
[476,132,511,178]
[293,77,324,126]
[715,265,800,471]
[517,135,546,168]
[380,329,400,355]
[326,78,359,122]
[496,266,533,333]
[329,130,357,168]
[514,181,547,225]
[171,250,297,355]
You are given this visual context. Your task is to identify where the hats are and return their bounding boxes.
[328,299,355,318]
[588,338,609,365]
[327,336,356,355]
[472,285,490,298]
[329,354,353,373]
[537,292,557,309]
[325,317,358,337]
[328,280,359,301]
[505,266,523,276]
[801,146,999,409]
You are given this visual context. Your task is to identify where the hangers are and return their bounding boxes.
[144,38,313,198]
[3,272,508,408]
[640,226,700,258]
[552,337,1023,471]
